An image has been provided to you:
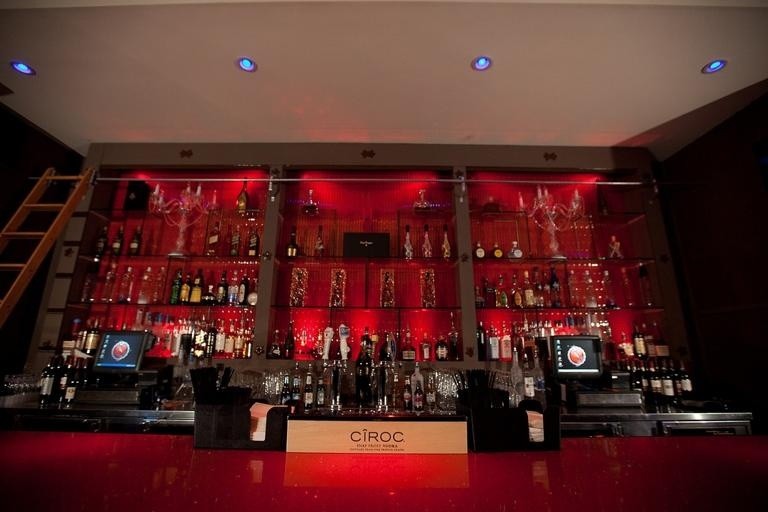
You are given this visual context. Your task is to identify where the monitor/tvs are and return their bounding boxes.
[92,331,150,372]
[550,335,604,376]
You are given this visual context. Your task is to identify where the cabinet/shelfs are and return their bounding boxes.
[264,143,465,377]
[465,146,698,369]
[47,143,269,361]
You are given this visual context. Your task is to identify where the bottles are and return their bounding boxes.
[83,316,101,354]
[207,221,219,257]
[508,240,523,256]
[402,225,415,258]
[607,234,624,258]
[237,177,248,210]
[128,225,143,255]
[94,225,108,254]
[118,265,135,302]
[411,361,424,406]
[488,319,498,360]
[189,269,203,304]
[82,357,92,377]
[215,319,225,352]
[153,265,167,303]
[272,330,280,356]
[655,330,672,357]
[500,319,513,358]
[491,241,502,258]
[484,277,494,307]
[40,358,55,406]
[413,380,425,410]
[402,376,413,409]
[217,270,228,305]
[248,227,260,255]
[304,372,314,407]
[231,225,242,254]
[641,323,655,356]
[476,286,484,307]
[238,270,249,304]
[533,356,544,401]
[359,326,372,360]
[583,270,598,306]
[138,264,153,303]
[425,378,436,409]
[550,268,560,307]
[510,351,521,406]
[299,319,309,346]
[601,270,615,308]
[434,336,447,360]
[541,271,550,306]
[477,320,487,361]
[414,189,432,210]
[301,189,319,213]
[633,325,646,356]
[448,312,459,360]
[497,274,509,307]
[228,273,238,305]
[293,375,301,400]
[522,270,534,306]
[206,320,217,346]
[421,224,432,256]
[474,241,485,257]
[619,359,693,397]
[312,222,326,256]
[64,357,81,401]
[130,309,174,357]
[282,376,290,404]
[514,311,613,360]
[380,333,394,360]
[170,268,182,304]
[179,272,191,304]
[418,333,432,361]
[243,334,252,358]
[399,331,415,360]
[79,260,95,301]
[53,358,72,404]
[568,270,579,304]
[509,276,522,306]
[521,355,534,400]
[441,223,452,258]
[285,225,299,256]
[77,319,87,352]
[317,375,326,405]
[618,333,634,357]
[235,320,244,349]
[99,264,119,300]
[285,321,294,358]
[203,285,216,304]
[225,320,235,352]
[532,266,544,305]
[111,226,125,255]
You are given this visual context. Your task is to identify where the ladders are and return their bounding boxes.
[0,167,97,328]
